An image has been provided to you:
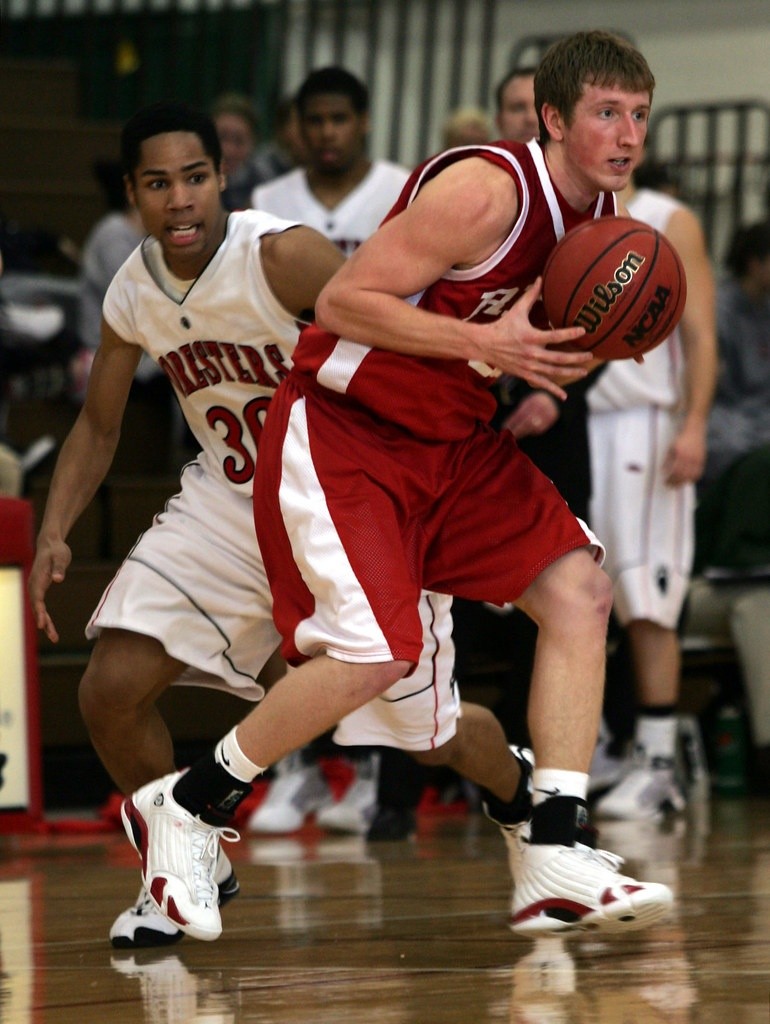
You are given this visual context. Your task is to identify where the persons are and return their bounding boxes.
[119,30,675,941]
[0,64,770,844]
[31,105,537,941]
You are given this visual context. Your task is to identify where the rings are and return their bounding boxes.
[531,416,541,426]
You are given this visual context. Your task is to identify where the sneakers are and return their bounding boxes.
[121,766,240,941]
[510,941,578,1024]
[111,956,237,1024]
[416,767,470,836]
[483,743,542,883]
[508,836,675,940]
[314,758,380,833]
[589,717,685,819]
[109,838,239,948]
[250,749,327,834]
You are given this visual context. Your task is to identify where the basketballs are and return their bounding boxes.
[539,215,690,361]
